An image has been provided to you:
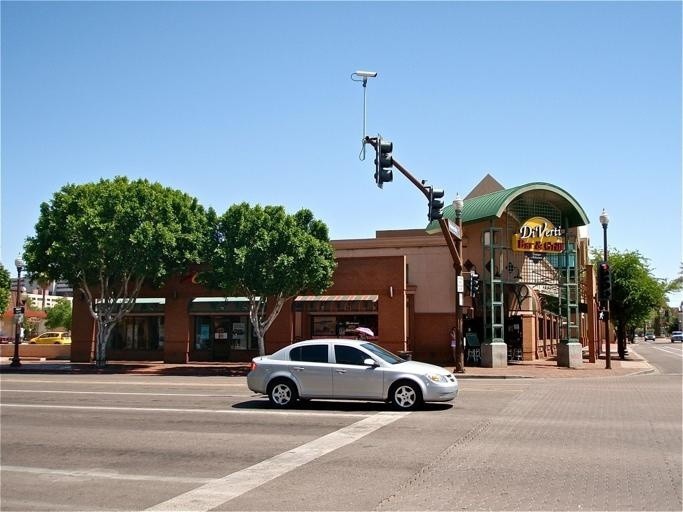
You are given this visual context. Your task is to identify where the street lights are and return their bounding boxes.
[451,193,464,374]
[600,207,610,368]
[10,257,23,367]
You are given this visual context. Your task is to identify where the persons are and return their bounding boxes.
[449,327,457,362]
[356,331,368,341]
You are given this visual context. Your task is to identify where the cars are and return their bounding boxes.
[671,331,682,342]
[644,332,655,341]
[246,337,458,410]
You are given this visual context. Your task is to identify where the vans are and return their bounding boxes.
[29,331,68,344]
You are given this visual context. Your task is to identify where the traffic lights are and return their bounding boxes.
[427,185,444,224]
[597,311,609,321]
[374,133,393,187]
[470,271,480,297]
[598,262,614,301]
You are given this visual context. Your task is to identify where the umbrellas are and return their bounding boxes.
[355,327,374,336]
